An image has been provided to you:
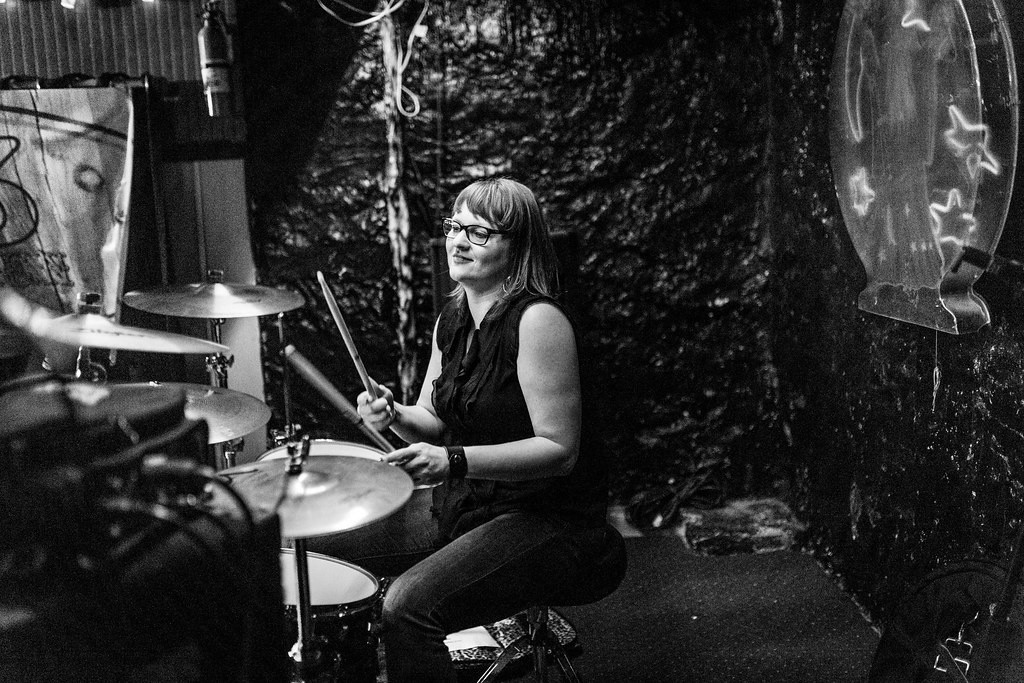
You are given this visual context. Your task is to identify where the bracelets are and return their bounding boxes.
[445,445,468,479]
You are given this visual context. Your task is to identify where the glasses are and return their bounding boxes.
[443,218,509,245]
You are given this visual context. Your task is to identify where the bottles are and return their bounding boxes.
[198,13,234,117]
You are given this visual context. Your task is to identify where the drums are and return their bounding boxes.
[280,548,380,683]
[256,439,395,468]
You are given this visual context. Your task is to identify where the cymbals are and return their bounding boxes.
[30,314,230,354]
[106,381,272,444]
[123,282,305,318]
[204,455,415,538]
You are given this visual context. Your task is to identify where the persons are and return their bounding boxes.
[305,179,609,683]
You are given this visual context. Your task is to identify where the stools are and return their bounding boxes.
[477,521,628,683]
